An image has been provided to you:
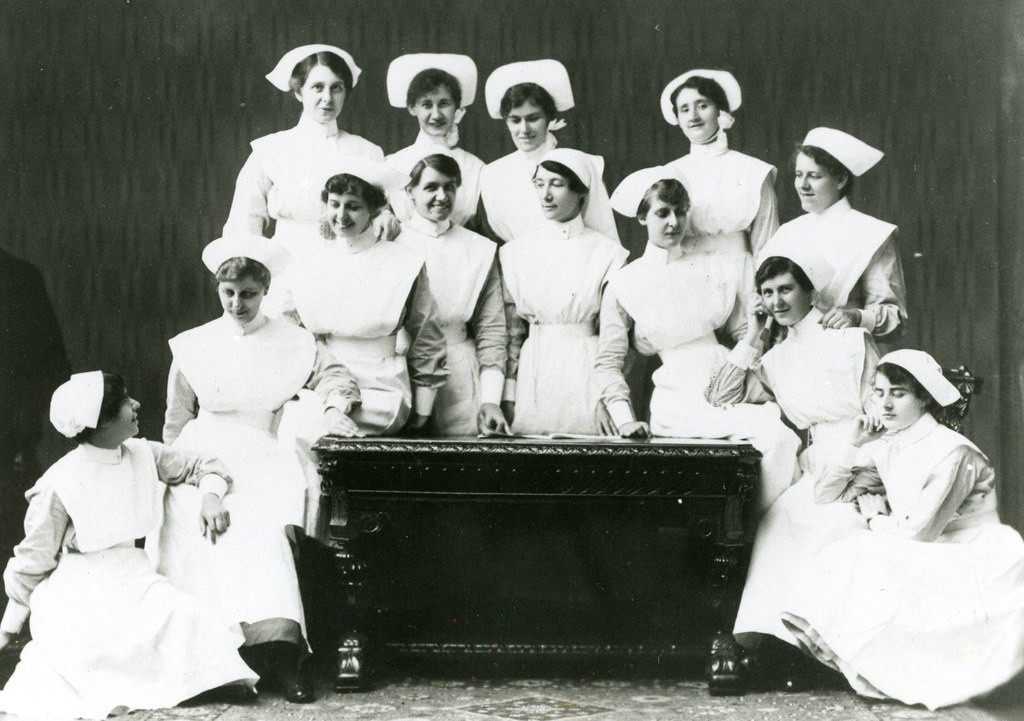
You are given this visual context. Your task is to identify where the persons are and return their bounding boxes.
[595,164,772,437]
[497,148,633,438]
[661,68,779,257]
[277,156,450,436]
[759,126,908,355]
[735,348,1024,710]
[224,45,402,241]
[155,237,364,703]
[384,53,486,229]
[0,369,262,721]
[701,248,882,472]
[476,59,620,245]
[393,146,512,435]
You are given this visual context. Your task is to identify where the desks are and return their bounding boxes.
[312,436,763,697]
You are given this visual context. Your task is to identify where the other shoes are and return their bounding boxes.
[277,662,316,703]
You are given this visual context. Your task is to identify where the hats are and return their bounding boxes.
[610,167,693,217]
[877,349,961,407]
[484,59,576,119]
[201,234,274,275]
[660,69,742,126]
[334,157,390,189]
[48,371,104,438]
[802,127,884,178]
[386,53,477,108]
[757,247,835,293]
[265,44,362,92]
[382,146,458,191]
